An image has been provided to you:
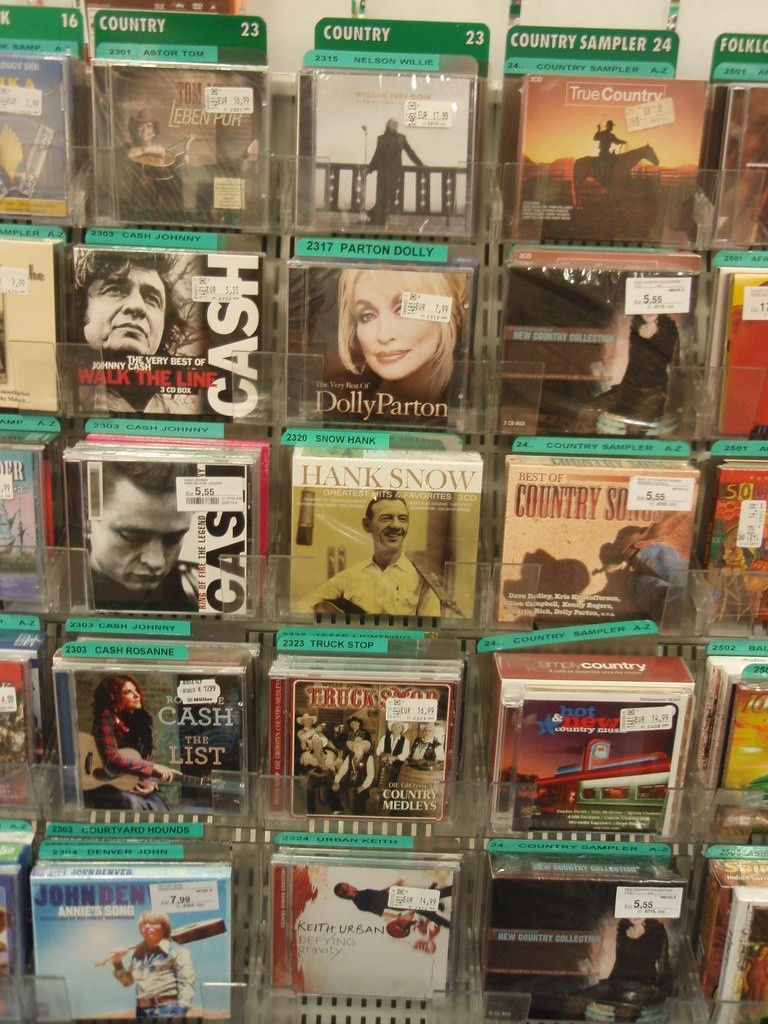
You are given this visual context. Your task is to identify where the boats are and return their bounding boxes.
[528,737,671,831]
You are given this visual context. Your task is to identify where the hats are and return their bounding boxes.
[346,737,371,754]
[297,714,317,725]
[345,716,363,728]
[387,720,408,733]
[420,722,435,734]
[128,109,156,135]
[604,120,616,127]
[305,733,328,748]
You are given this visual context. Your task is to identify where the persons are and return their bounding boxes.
[84,674,183,811]
[113,106,627,225]
[568,315,681,436]
[111,910,195,1018]
[333,879,452,928]
[337,268,471,434]
[74,248,205,415]
[298,495,441,617]
[84,463,199,611]
[564,918,669,1024]
[613,511,690,623]
[296,712,445,816]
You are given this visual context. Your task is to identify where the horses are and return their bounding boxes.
[570,142,660,207]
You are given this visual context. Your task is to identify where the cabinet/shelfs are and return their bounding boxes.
[0,0,768,1024]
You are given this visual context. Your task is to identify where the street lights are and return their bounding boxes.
[361,125,368,163]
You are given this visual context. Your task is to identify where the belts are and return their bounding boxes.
[137,997,177,1008]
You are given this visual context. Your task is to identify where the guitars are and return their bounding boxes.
[365,758,392,816]
[130,134,191,182]
[385,880,438,939]
[92,917,228,969]
[77,730,226,793]
